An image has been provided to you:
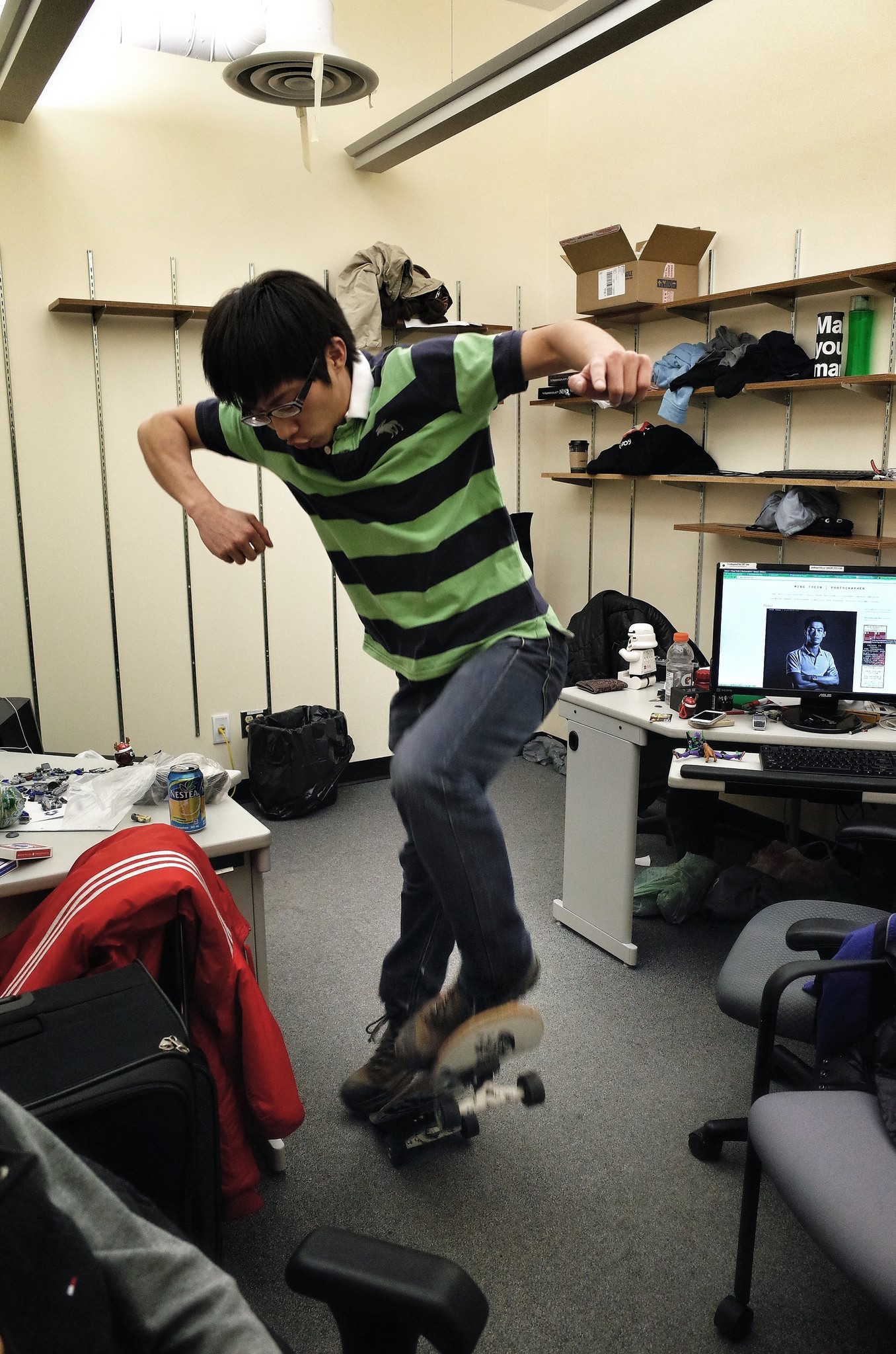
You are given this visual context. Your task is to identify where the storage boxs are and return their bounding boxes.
[559,220,717,316]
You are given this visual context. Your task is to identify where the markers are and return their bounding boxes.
[768,715,780,721]
[749,698,774,708]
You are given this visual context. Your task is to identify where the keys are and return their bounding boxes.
[649,694,665,702]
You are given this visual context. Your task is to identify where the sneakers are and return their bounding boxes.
[390,951,541,1070]
[337,998,447,1115]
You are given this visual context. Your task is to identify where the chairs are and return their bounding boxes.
[685,821,896,1164]
[712,955,896,1336]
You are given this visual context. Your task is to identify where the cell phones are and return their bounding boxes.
[688,709,727,725]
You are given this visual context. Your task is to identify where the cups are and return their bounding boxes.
[568,440,589,474]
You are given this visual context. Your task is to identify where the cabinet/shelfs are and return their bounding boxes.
[50,258,896,554]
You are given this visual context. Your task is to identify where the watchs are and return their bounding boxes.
[811,674,817,683]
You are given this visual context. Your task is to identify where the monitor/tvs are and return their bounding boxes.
[711,561,896,735]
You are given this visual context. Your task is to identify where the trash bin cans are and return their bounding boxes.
[247,704,355,819]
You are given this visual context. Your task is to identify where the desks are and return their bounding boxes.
[546,677,896,969]
[2,752,272,1023]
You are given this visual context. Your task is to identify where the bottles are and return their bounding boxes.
[844,295,876,377]
[665,633,694,705]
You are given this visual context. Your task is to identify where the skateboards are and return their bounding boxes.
[367,1001,546,1164]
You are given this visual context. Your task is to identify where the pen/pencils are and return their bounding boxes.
[747,710,755,715]
[849,722,878,735]
[724,710,749,715]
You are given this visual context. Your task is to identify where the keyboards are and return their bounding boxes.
[760,743,896,779]
[756,468,894,481]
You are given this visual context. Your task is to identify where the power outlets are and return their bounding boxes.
[240,709,269,739]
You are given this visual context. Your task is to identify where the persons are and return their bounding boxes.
[672,731,745,762]
[138,270,655,1112]
[786,615,839,690]
[612,623,658,690]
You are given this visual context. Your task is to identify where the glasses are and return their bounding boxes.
[233,347,323,428]
